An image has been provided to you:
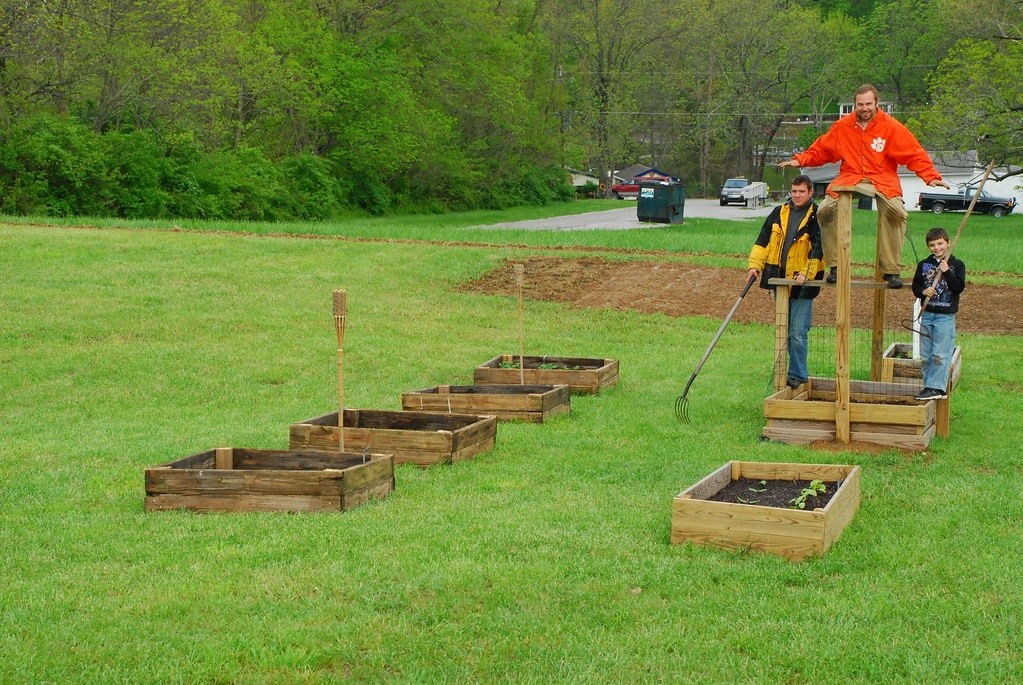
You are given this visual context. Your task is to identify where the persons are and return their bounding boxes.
[779,85,950,289]
[743,176,826,390]
[911,227,966,400]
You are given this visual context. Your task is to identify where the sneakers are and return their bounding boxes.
[914,387,947,400]
[882,273,904,289]
[787,376,809,389]
[826,267,837,284]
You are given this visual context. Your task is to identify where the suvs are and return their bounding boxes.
[719,178,748,206]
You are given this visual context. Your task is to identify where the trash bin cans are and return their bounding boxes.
[636,181,686,225]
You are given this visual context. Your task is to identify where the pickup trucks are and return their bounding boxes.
[610,176,670,200]
[918,186,1016,218]
